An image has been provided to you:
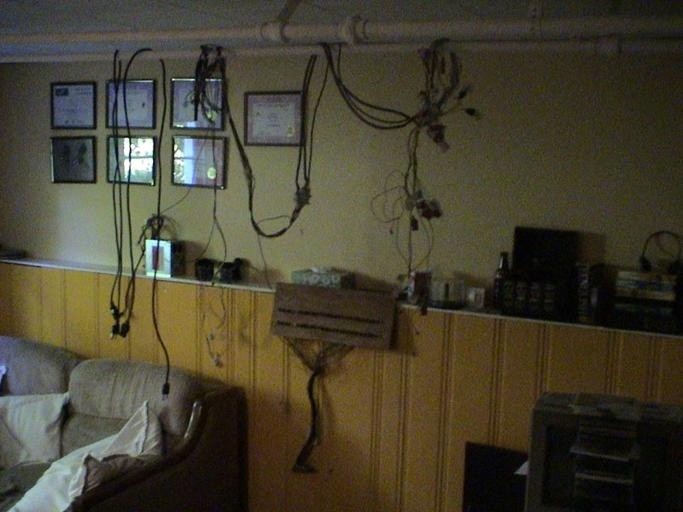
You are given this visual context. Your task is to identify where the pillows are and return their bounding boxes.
[8,400,164,512]
[0,389,72,465]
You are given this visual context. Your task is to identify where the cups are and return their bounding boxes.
[466,285,486,308]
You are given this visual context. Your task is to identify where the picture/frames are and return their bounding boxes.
[50,136,98,184]
[103,79,158,129]
[244,89,305,147]
[105,134,158,186]
[170,134,229,190]
[49,81,98,129]
[169,77,227,131]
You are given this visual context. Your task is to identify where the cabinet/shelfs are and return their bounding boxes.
[524,390,683,512]
[0,262,683,511]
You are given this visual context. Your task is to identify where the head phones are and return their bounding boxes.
[639,232,682,276]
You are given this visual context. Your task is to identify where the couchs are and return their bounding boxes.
[0,334,250,512]
